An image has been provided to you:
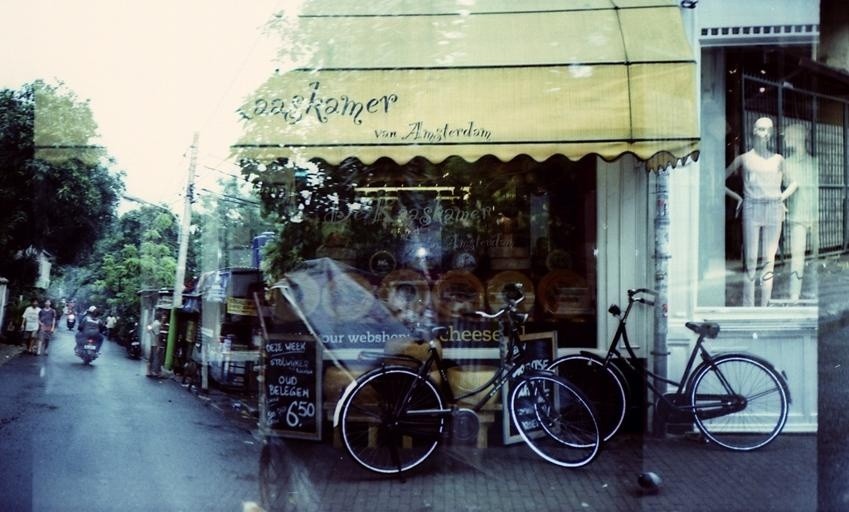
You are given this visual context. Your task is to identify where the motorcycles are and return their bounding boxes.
[66,313,75,331]
[74,334,101,364]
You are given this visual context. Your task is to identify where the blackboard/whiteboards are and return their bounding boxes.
[258,334,321,441]
[499,330,561,445]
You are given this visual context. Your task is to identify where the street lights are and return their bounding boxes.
[122,192,194,375]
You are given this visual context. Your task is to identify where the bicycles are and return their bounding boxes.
[330,283,602,473]
[532,287,792,454]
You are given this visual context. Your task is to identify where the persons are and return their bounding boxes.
[22,296,42,355]
[60,296,142,362]
[161,310,171,346]
[778,122,818,304]
[725,116,800,306]
[0,318,23,347]
[147,310,165,377]
[34,298,57,356]
